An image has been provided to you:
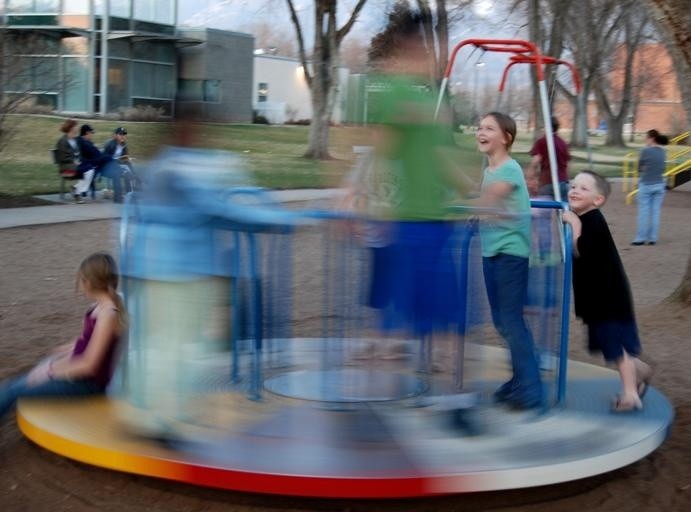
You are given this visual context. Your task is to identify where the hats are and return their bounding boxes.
[116,127,127,134]
[80,124,92,134]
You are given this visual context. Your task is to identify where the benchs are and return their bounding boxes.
[51,146,137,201]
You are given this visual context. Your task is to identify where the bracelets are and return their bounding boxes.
[47,365,55,381]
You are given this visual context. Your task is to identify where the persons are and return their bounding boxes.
[78,125,123,204]
[0,249,131,417]
[119,79,476,450]
[105,128,144,192]
[631,129,666,247]
[527,115,572,263]
[561,171,654,415]
[452,110,546,413]
[56,119,95,204]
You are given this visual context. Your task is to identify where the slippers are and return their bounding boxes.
[611,365,654,413]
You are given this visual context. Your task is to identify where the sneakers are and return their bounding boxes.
[73,194,83,203]
